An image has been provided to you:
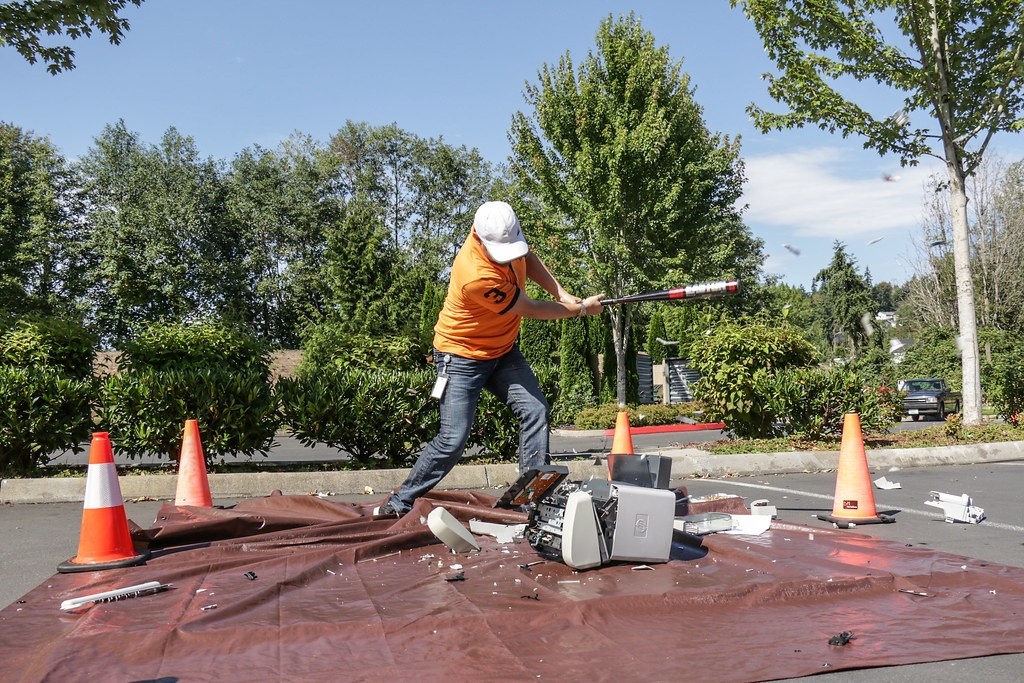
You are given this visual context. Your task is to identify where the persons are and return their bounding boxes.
[378,199,606,519]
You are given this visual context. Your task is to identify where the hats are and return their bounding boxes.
[474,200,529,265]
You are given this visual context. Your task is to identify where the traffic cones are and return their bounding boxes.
[175,420,236,510]
[818,412,898,527]
[56,431,155,572]
[607,413,635,479]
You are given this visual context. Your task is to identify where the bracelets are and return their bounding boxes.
[576,299,587,318]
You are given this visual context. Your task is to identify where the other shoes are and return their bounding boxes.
[373,496,400,516]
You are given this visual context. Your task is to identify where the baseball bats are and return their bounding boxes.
[598,277,741,306]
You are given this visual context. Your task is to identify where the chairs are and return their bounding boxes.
[914,386,920,391]
[933,383,941,389]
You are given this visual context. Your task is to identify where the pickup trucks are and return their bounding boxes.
[891,378,962,422]
